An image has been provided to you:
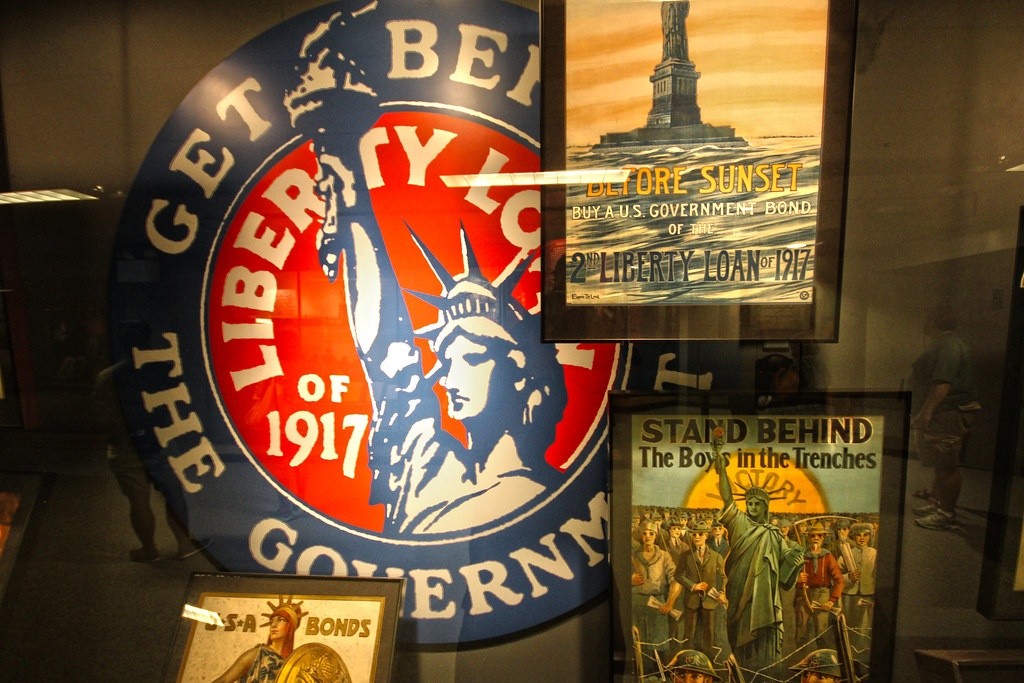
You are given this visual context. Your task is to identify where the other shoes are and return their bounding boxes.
[128,546,160,563]
[180,535,215,560]
[911,489,931,499]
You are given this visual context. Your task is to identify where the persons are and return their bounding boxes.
[94,319,215,561]
[265,490,293,522]
[904,308,986,531]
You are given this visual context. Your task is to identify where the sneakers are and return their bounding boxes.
[915,509,958,530]
[912,501,942,518]
